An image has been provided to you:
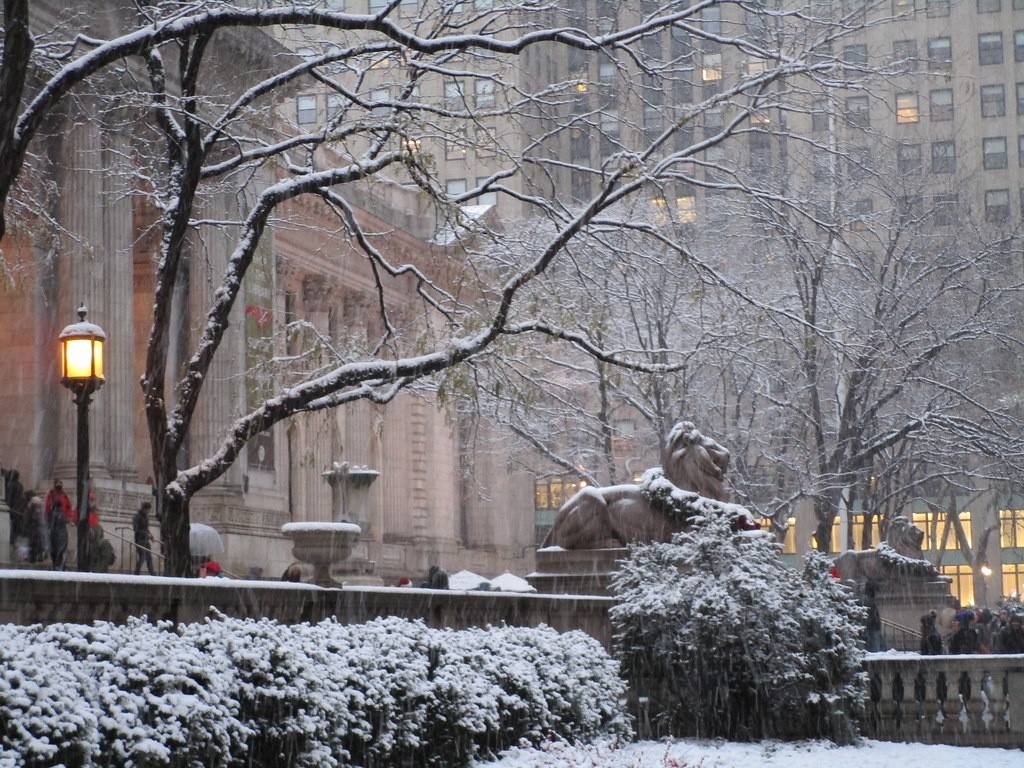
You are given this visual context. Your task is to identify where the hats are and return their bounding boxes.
[55,479,64,488]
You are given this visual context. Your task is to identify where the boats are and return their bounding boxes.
[58,301,108,570]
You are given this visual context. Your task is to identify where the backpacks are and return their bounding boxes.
[104,541,117,567]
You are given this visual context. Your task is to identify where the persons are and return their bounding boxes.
[424,566,449,590]
[191,554,224,579]
[856,585,1024,719]
[1,463,116,575]
[132,501,155,575]
[397,578,413,588]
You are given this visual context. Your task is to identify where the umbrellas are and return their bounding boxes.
[446,568,501,591]
[189,522,225,557]
[489,570,539,592]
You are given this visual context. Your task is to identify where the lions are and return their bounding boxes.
[540,418,776,550]
[834,515,956,585]
